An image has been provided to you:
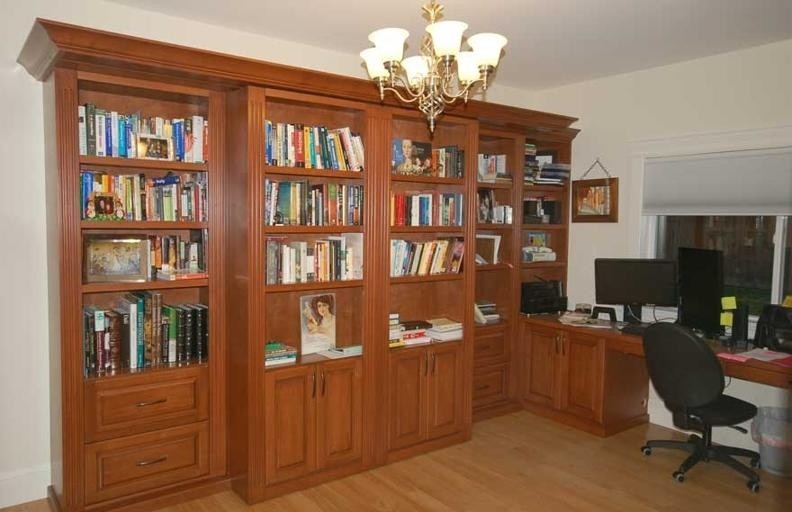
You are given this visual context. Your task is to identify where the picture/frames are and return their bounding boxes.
[573,176,620,222]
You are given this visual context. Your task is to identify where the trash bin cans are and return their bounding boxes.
[753,407,792,478]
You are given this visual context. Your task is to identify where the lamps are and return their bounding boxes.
[359,0,507,130]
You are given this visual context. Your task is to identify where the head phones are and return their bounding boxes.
[719,338,748,354]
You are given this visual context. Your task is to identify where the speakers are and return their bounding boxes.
[679,248,726,340]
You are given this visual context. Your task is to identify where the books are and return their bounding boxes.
[298,293,336,356]
[265,342,297,370]
[475,152,513,265]
[521,143,572,264]
[557,308,613,330]
[262,119,364,285]
[390,137,465,278]
[475,302,500,325]
[388,312,463,348]
[317,345,364,360]
[78,102,209,372]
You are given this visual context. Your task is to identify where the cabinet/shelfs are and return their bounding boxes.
[15,17,580,512]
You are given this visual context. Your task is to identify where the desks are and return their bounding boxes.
[522,312,792,438]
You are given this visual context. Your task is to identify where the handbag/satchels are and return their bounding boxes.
[754,305,792,351]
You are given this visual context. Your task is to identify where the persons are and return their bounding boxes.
[303,295,335,342]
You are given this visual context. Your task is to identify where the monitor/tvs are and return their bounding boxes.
[594,258,679,306]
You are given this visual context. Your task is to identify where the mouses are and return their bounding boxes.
[618,324,625,330]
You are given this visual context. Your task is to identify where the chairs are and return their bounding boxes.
[641,322,760,492]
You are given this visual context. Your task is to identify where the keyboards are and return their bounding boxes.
[621,327,647,336]
